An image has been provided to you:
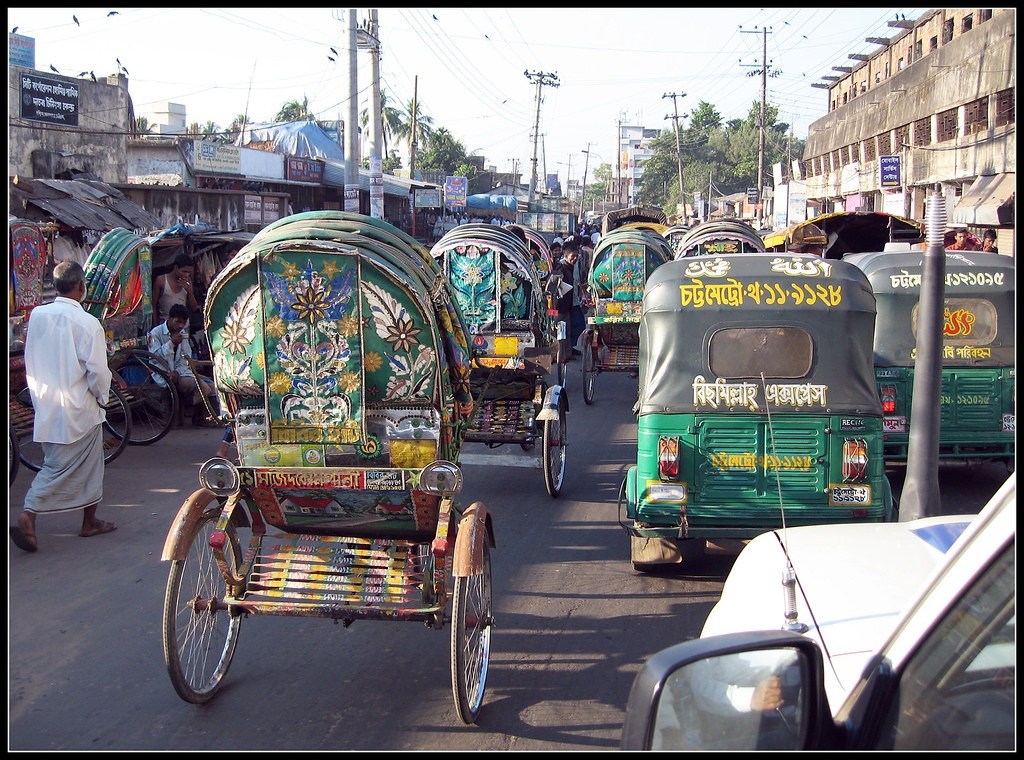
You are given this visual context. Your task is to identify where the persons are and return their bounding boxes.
[686,215,694,228]
[148,304,220,427]
[549,232,594,364]
[429,209,516,243]
[10,260,117,552]
[505,225,526,243]
[211,240,244,281]
[152,253,197,329]
[575,220,602,248]
[971,229,998,254]
[945,228,972,251]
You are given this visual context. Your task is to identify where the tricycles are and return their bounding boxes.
[161,211,572,705]
[11,214,177,485]
[582,206,983,406]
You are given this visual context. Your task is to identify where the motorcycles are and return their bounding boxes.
[623,252,1013,572]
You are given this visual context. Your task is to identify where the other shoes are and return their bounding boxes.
[572,349,581,355]
[569,356,576,360]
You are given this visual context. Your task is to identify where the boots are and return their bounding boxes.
[192,401,216,427]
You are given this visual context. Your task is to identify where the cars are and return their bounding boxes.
[627,458,1013,752]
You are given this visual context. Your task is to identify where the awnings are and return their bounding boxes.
[953,172,1015,225]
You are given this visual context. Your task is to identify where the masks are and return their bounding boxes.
[78,284,87,304]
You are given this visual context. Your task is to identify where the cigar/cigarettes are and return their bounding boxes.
[186,282,189,284]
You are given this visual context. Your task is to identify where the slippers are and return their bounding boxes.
[79,519,117,537]
[10,526,38,552]
